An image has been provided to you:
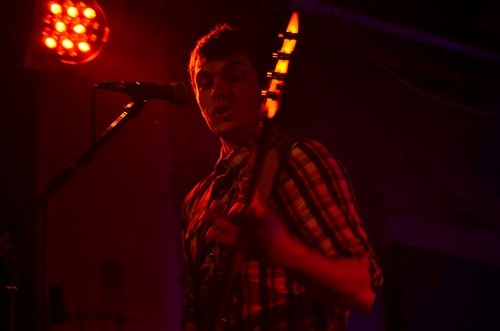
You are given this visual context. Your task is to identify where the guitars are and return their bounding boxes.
[191,11,302,331]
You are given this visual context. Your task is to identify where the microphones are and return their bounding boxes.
[98,79,192,108]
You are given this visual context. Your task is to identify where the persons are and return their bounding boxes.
[175,26,383,331]
